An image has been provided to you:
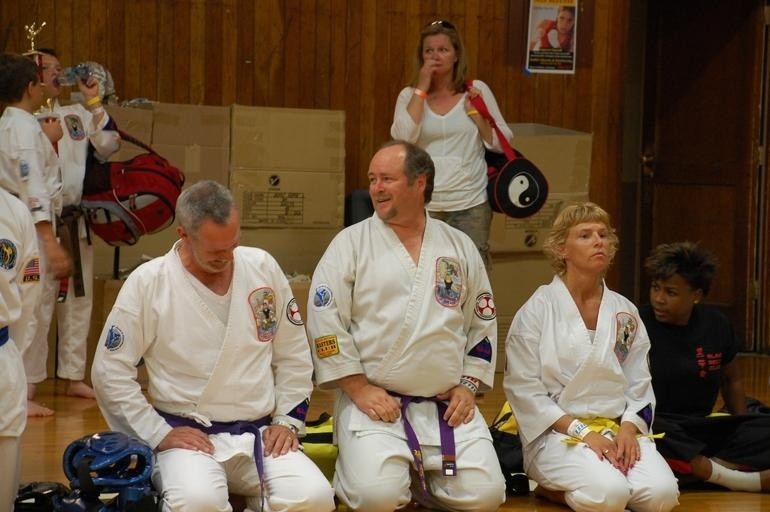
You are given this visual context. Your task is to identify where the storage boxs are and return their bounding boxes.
[38,96,597,392]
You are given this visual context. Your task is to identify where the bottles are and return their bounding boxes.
[57,63,88,87]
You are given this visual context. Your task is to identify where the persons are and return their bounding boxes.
[307,139,506,512]
[503,201,681,512]
[390,17,514,273]
[639,240,770,493]
[531,5,576,51]
[91,180,336,512]
[0,49,123,512]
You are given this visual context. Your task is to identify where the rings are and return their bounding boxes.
[605,449,609,454]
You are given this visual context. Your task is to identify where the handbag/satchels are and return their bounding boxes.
[81,152,184,247]
[485,147,549,219]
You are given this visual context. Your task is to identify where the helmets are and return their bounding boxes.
[16,431,158,511]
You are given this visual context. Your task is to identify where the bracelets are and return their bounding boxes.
[414,88,428,98]
[567,419,593,440]
[460,376,480,395]
